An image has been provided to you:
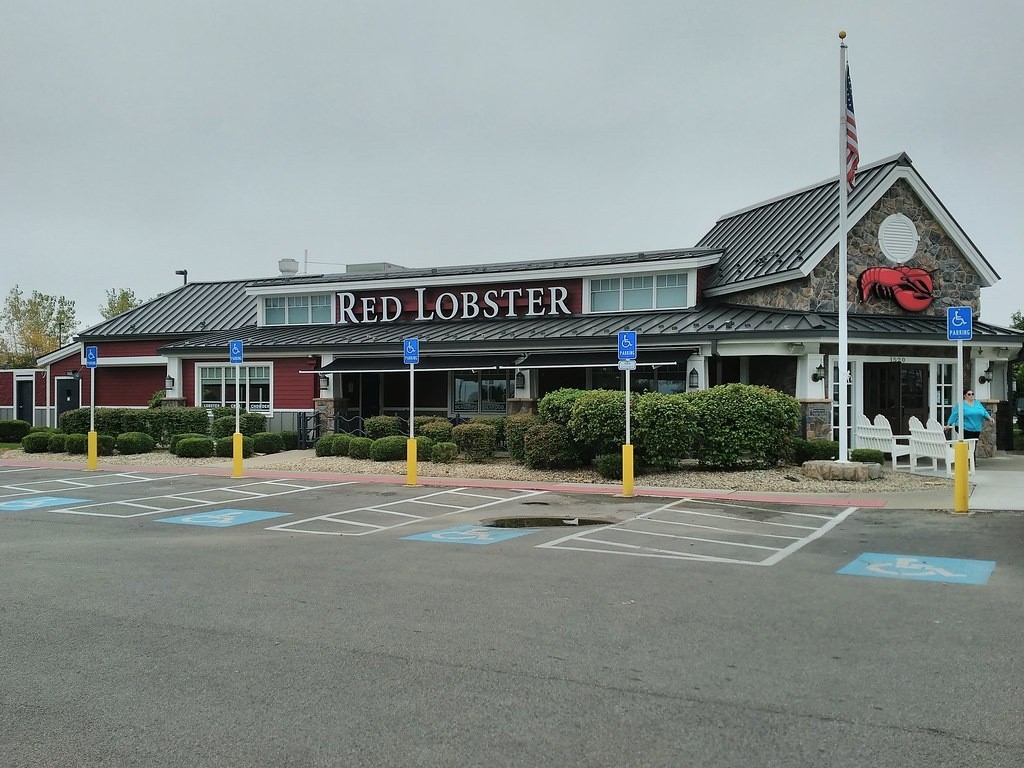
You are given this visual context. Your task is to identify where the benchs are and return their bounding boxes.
[854,413,917,471]
[907,415,980,478]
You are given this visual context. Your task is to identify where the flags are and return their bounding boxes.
[842,50,860,197]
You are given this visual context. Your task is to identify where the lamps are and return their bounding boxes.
[979,366,993,384]
[516,372,525,389]
[811,364,825,382]
[1011,377,1017,392]
[689,368,698,388]
[164,375,174,390]
[319,374,329,390]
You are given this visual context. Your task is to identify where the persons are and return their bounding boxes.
[942,389,995,470]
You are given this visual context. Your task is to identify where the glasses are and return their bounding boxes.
[965,393,974,396]
